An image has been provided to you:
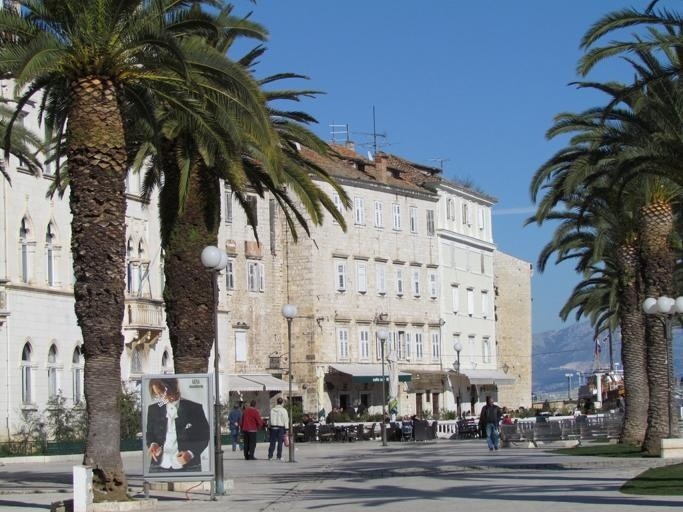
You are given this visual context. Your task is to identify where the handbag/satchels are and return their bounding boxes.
[282,434,291,448]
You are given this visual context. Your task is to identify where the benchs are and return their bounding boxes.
[498,416,624,444]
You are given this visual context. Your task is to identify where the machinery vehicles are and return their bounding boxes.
[576,372,624,414]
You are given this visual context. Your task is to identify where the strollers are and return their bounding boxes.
[399,415,414,444]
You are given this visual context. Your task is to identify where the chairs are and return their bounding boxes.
[292,419,438,443]
[454,417,481,440]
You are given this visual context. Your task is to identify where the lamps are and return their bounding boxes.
[499,362,509,375]
[453,361,460,372]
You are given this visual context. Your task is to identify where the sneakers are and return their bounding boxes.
[488,446,499,451]
[231,448,285,462]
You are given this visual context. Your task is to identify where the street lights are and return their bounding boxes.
[641,295,682,459]
[564,372,573,401]
[201,240,231,497]
[281,302,299,464]
[451,339,464,418]
[376,325,391,447]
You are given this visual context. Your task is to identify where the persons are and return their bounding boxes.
[480,396,501,450]
[268,398,289,460]
[412,415,420,421]
[146,378,210,472]
[326,402,366,428]
[241,400,268,459]
[228,404,244,451]
[302,414,312,425]
